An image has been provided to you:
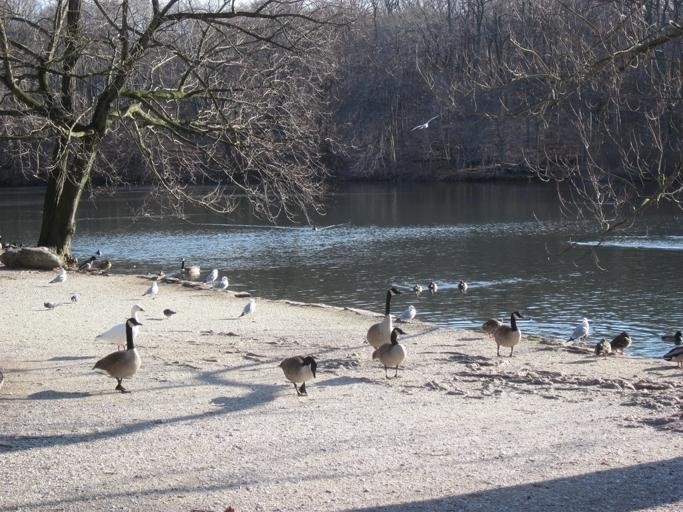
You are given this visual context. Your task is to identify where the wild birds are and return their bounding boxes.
[163,308,177,319]
[275,351,317,396]
[77,248,113,275]
[594,331,631,361]
[177,257,229,292]
[458,280,468,295]
[49,269,69,285]
[142,281,160,299]
[413,284,422,296]
[564,317,590,344]
[86,303,146,395]
[662,330,683,368]
[43,301,57,309]
[481,309,526,359]
[428,281,438,296]
[237,297,259,323]
[364,286,418,382]
